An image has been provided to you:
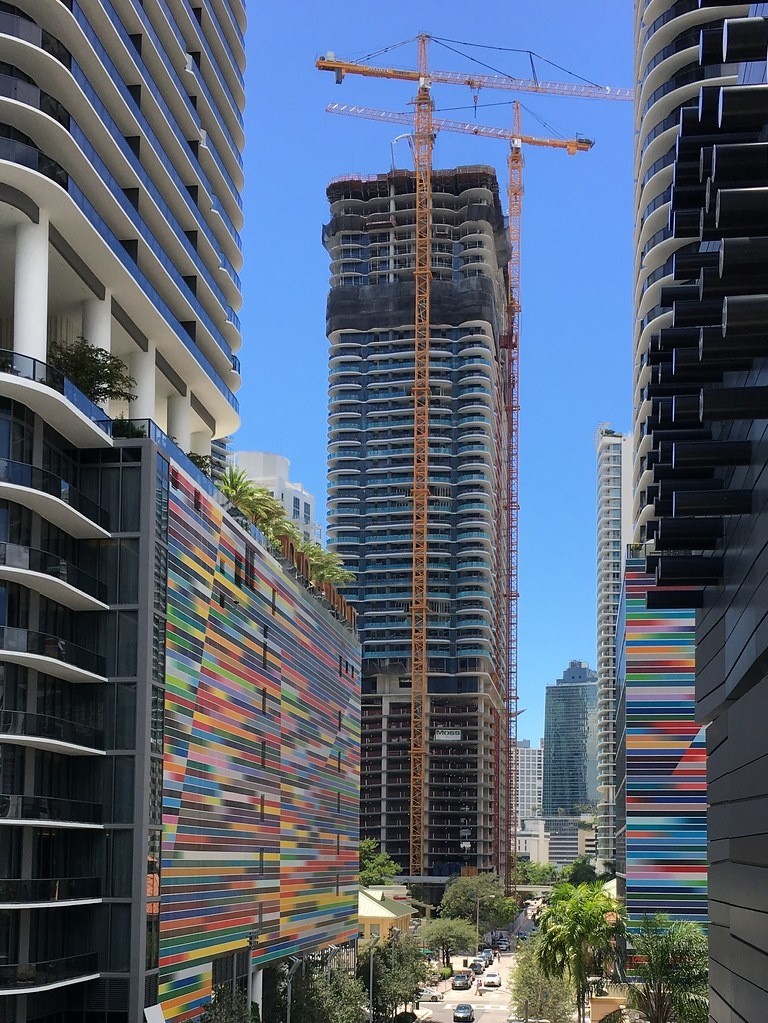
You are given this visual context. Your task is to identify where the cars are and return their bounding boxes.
[484,972,501,987]
[415,987,444,1002]
[452,1004,474,1022]
[499,938,510,950]
[497,941,507,951]
[469,963,483,974]
[462,969,476,981]
[474,943,494,971]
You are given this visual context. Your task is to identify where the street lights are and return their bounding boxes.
[409,919,421,1009]
[369,932,380,1023]
[285,955,302,1023]
[327,944,340,983]
[476,894,495,955]
[388,927,402,973]
[478,988,528,1023]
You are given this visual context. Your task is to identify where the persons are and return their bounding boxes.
[475,979,483,996]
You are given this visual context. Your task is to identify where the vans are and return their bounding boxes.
[516,932,526,941]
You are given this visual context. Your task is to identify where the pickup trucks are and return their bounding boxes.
[452,974,472,990]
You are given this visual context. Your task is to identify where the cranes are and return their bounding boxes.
[314,32,635,876]
[328,96,597,893]
